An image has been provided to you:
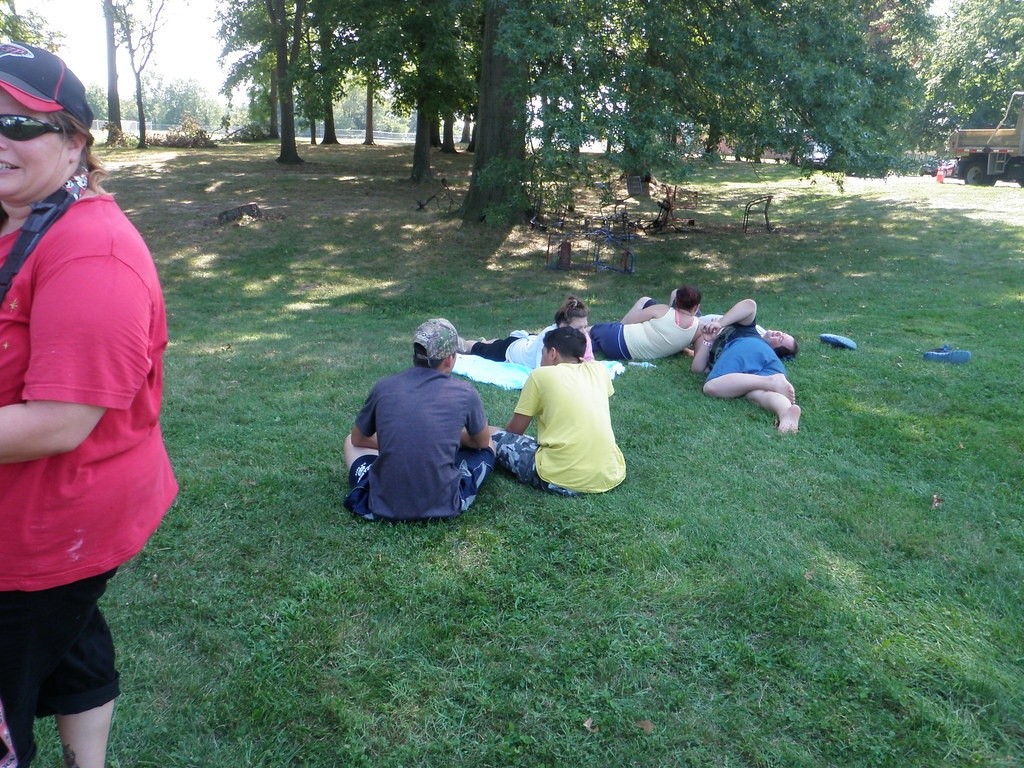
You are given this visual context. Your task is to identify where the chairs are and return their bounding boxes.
[742,195,774,234]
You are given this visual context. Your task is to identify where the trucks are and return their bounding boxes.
[945,90,1024,188]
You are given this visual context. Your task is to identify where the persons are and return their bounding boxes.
[464,296,596,368]
[342,319,496,522]
[619,297,798,357]
[580,287,703,361]
[0,42,178,766]
[691,299,802,434]
[486,327,626,497]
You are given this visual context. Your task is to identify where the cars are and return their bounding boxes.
[919,156,957,177]
[795,142,830,169]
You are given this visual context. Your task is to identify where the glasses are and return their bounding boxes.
[0,113,74,143]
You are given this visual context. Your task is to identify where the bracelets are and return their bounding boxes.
[703,340,713,347]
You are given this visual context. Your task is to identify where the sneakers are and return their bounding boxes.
[923,345,972,363]
[818,332,857,349]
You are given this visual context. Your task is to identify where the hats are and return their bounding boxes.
[0,42,95,129]
[413,317,468,362]
